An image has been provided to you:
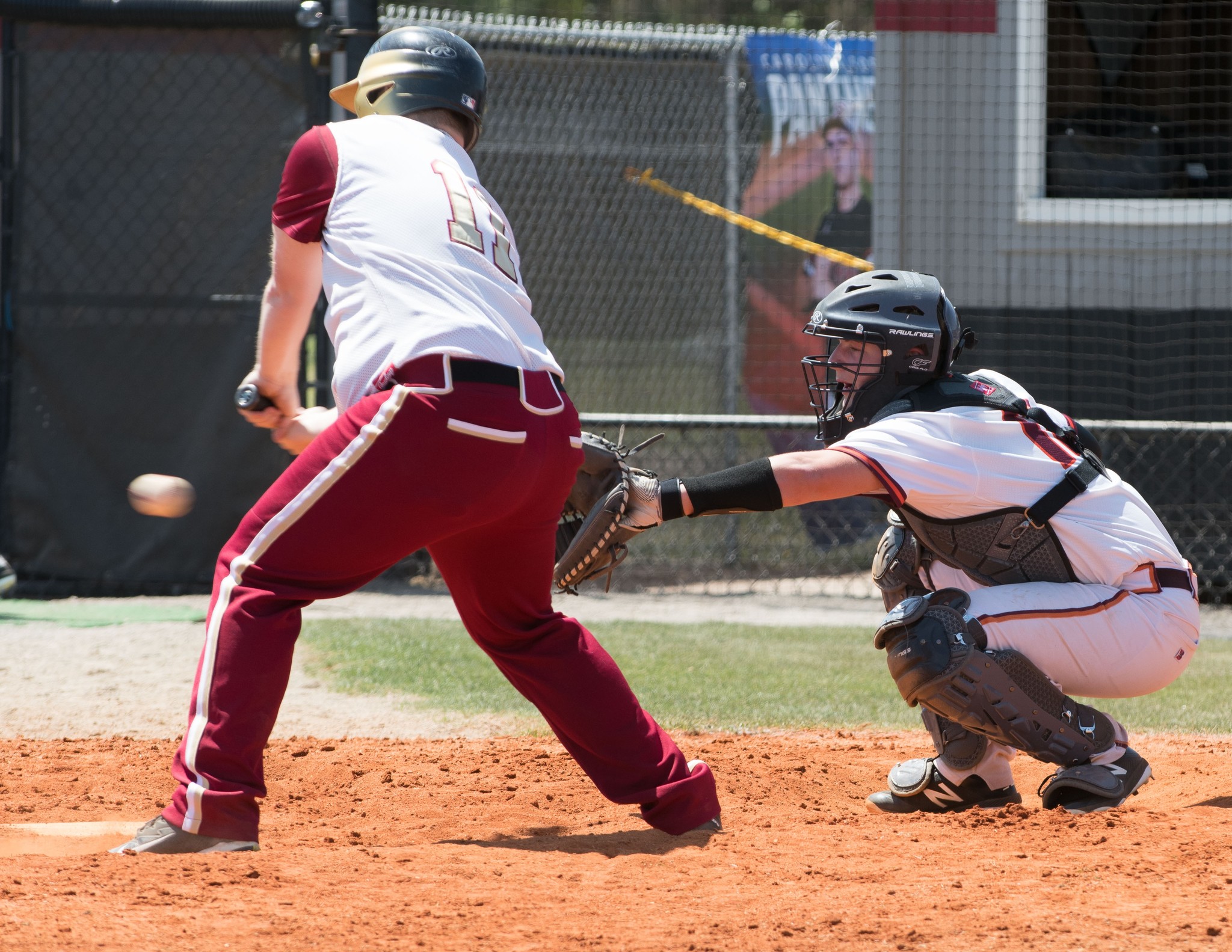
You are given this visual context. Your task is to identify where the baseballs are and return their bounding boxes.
[125,473,195,519]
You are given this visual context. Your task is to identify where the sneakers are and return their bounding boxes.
[1058,747,1154,815]
[108,816,260,854]
[865,769,1022,814]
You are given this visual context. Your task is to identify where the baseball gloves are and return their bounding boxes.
[551,421,665,597]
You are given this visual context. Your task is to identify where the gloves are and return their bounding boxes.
[619,472,662,528]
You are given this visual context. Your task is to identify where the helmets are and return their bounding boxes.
[802,270,961,444]
[329,26,487,152]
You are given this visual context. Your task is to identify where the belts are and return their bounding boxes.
[1156,568,1193,592]
[450,359,566,391]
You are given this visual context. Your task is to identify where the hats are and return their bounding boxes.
[823,117,856,135]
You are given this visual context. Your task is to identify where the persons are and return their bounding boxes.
[803,117,872,311]
[112,25,725,860]
[620,270,1198,813]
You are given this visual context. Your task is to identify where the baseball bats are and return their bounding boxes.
[233,382,331,431]
